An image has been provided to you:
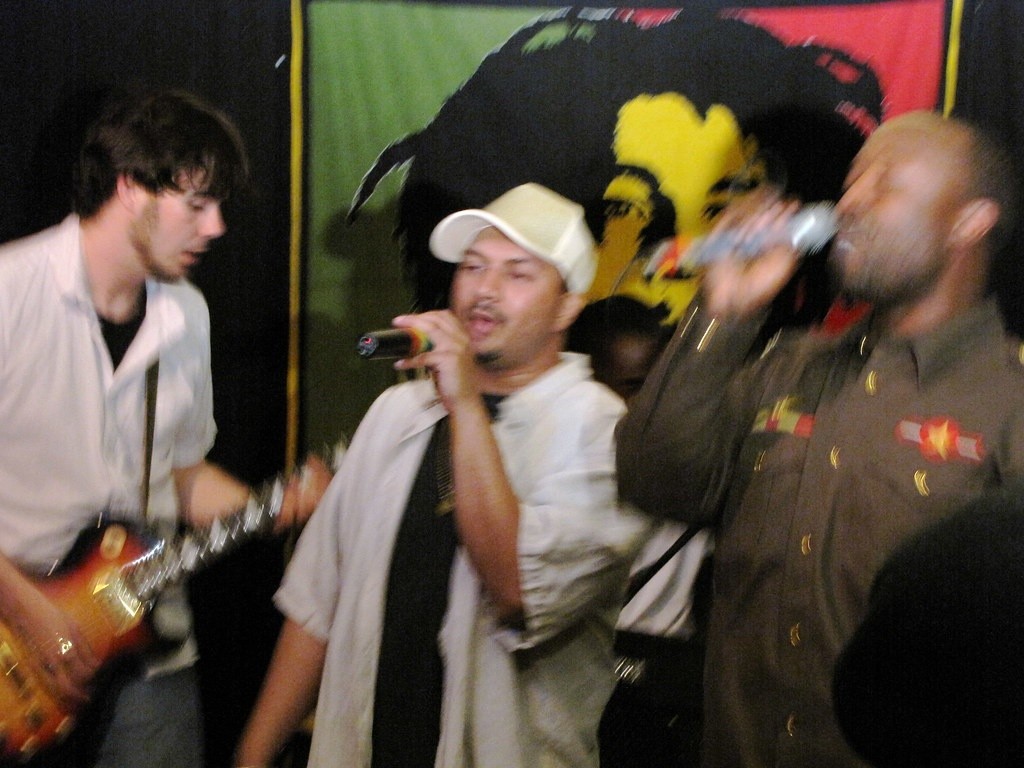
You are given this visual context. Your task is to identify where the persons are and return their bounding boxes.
[236,184,651,768]
[1,88,332,768]
[615,111,1024,768]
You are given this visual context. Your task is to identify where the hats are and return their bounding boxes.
[429,184,599,295]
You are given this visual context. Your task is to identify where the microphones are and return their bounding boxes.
[646,200,836,276]
[357,328,434,361]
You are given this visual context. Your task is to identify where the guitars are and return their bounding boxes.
[0,428,367,768]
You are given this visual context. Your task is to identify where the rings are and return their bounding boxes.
[58,642,71,655]
